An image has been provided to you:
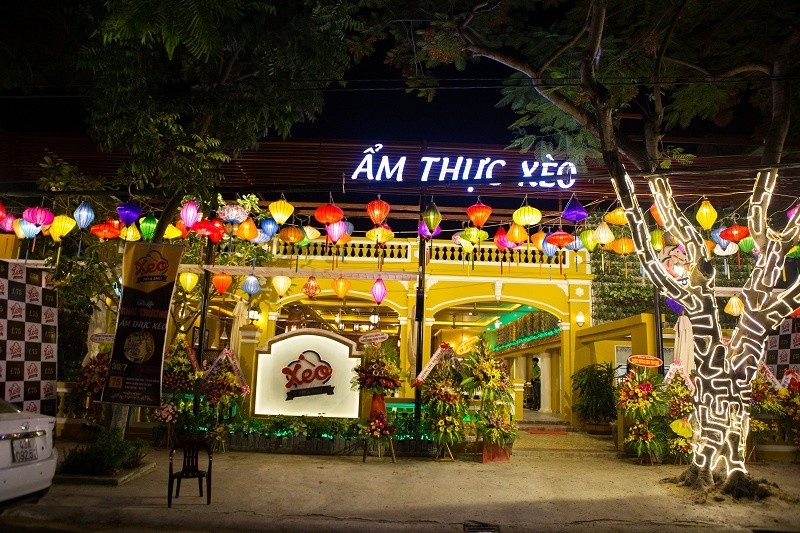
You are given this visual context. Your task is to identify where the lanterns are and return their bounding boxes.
[0,188,800,332]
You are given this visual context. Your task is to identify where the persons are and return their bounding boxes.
[530,357,541,411]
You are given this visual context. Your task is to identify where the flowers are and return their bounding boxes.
[72,339,251,454]
[749,366,800,442]
[350,332,520,447]
[614,359,697,466]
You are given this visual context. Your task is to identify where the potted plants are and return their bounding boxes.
[571,361,621,434]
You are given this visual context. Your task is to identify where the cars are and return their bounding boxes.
[1,399,60,514]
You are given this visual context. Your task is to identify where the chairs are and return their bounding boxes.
[168,438,213,508]
